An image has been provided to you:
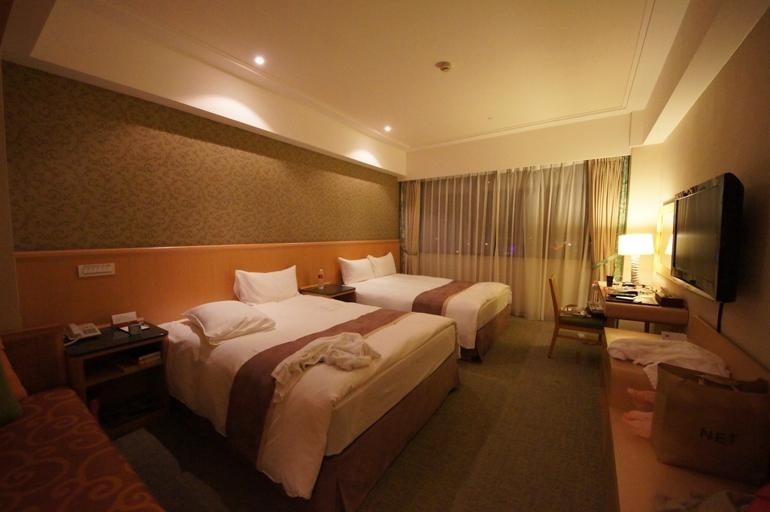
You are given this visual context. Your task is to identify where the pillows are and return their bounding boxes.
[366,251,396,277]
[337,257,375,285]
[183,300,276,346]
[233,264,299,306]
[0,342,28,402]
[0,362,24,429]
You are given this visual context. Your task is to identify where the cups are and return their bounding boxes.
[607,276,614,288]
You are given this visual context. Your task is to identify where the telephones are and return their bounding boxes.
[64,323,102,347]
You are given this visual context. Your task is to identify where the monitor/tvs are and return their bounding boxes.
[670,172,746,305]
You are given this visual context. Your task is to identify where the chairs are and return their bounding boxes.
[548,272,604,369]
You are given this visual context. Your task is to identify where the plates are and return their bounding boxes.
[616,296,633,301]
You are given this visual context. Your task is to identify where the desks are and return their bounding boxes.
[597,280,688,333]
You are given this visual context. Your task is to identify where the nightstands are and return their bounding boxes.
[298,283,356,304]
[63,320,169,442]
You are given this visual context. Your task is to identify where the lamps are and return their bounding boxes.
[617,233,655,285]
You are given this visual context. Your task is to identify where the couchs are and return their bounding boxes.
[0,340,165,511]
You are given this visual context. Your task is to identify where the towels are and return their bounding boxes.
[607,338,730,390]
[270,332,382,404]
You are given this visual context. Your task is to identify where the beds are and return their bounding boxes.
[341,273,512,362]
[157,294,462,512]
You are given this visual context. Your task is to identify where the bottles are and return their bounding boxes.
[318,269,324,290]
